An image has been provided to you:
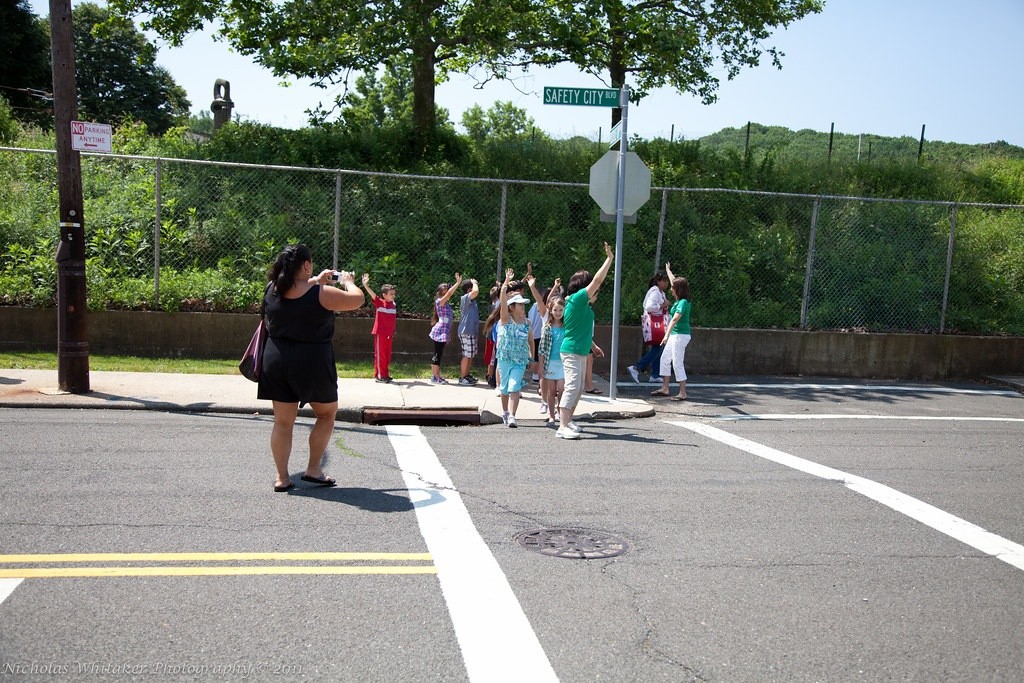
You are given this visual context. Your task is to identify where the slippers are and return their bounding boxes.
[300,473,336,485]
[586,387,603,394]
[671,395,686,401]
[274,479,294,492]
[650,390,670,396]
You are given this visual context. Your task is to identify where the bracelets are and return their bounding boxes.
[313,276,319,285]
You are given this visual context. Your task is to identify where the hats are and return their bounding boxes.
[506,294,531,306]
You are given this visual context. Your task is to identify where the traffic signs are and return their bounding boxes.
[609,120,621,148]
[71,121,112,152]
[544,86,621,108]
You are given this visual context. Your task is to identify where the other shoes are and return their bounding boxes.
[430,374,449,384]
[458,373,477,385]
[648,376,664,382]
[375,376,393,383]
[627,366,639,384]
[502,411,518,428]
[555,427,580,439]
[531,376,540,383]
[546,418,555,429]
[540,401,548,413]
[486,373,492,385]
[568,421,583,432]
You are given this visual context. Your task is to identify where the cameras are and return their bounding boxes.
[331,272,342,282]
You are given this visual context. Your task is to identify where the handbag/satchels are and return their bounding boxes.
[641,313,670,346]
[239,319,267,382]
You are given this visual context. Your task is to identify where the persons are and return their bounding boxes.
[627,271,669,384]
[257,244,365,491]
[483,262,565,388]
[583,288,603,395]
[362,272,396,383]
[538,296,604,427]
[495,268,535,428]
[458,279,479,386]
[429,272,463,385]
[556,241,614,439]
[649,262,691,401]
